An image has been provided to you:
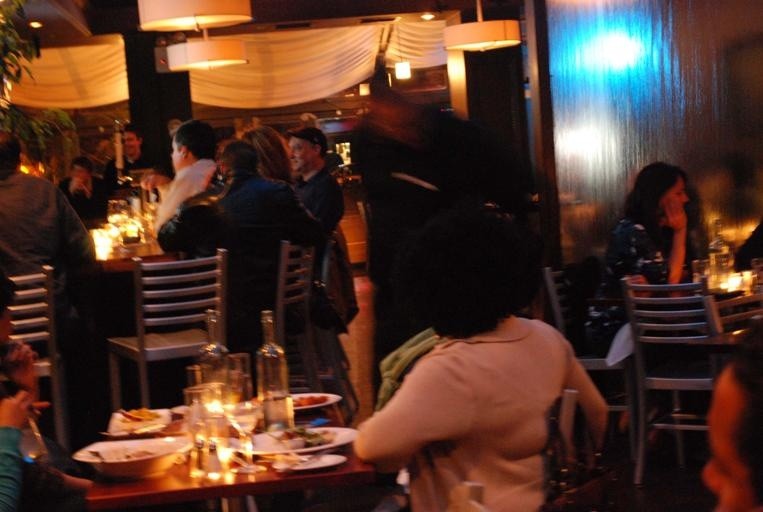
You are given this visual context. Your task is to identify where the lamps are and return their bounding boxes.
[418,3,435,22]
[138,0,253,32]
[393,16,414,81]
[354,81,370,100]
[440,1,522,52]
[165,27,251,72]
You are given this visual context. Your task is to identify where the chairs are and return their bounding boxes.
[250,239,323,394]
[542,262,637,467]
[619,275,725,487]
[0,264,72,462]
[104,247,228,415]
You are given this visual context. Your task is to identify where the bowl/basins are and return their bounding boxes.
[71,435,195,480]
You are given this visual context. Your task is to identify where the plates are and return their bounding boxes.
[290,392,344,411]
[252,426,359,459]
[271,454,348,472]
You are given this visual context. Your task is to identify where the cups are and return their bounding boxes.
[226,353,254,404]
[121,227,141,245]
[187,365,218,383]
[106,200,129,227]
[690,259,712,290]
[751,258,761,294]
[182,383,228,485]
[222,404,267,473]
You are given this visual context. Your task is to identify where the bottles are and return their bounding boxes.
[258,310,294,433]
[709,219,730,294]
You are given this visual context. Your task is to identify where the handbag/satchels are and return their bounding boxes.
[535,393,622,511]
[311,237,352,335]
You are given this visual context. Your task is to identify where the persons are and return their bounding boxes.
[0,132,95,333]
[59,156,105,220]
[1,272,49,406]
[157,120,184,171]
[583,161,690,438]
[160,119,221,225]
[284,125,347,231]
[243,126,292,182]
[102,122,144,189]
[159,141,324,311]
[702,319,762,512]
[352,197,609,512]
[1,389,35,511]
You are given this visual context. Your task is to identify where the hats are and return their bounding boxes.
[287,127,328,152]
